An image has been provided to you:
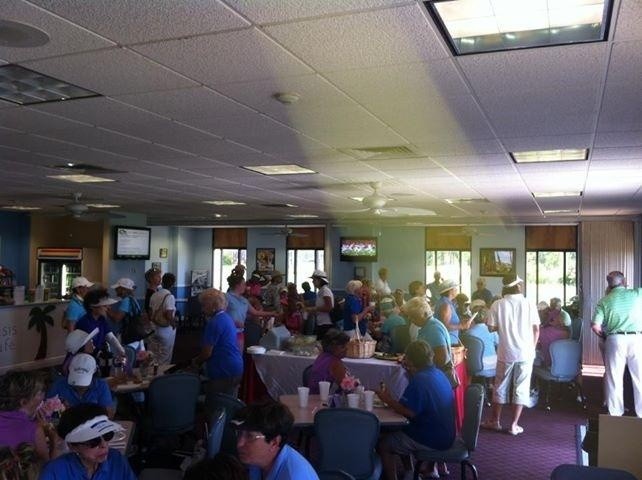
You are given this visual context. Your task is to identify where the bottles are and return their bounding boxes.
[67,264,81,287]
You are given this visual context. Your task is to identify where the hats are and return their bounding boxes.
[235,264,281,278]
[89,296,119,307]
[309,269,329,284]
[67,352,98,387]
[63,413,121,445]
[438,278,462,294]
[64,327,100,355]
[111,278,134,290]
[71,276,95,289]
[505,275,524,288]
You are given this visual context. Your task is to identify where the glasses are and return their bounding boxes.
[78,431,114,449]
[236,434,268,441]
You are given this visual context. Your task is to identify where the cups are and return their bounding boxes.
[318,381,331,400]
[363,390,375,412]
[298,386,310,408]
[345,392,361,408]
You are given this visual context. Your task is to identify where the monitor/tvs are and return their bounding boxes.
[114,225,151,259]
[339,236,378,262]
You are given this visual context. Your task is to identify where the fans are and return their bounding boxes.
[259,223,309,237]
[30,193,126,218]
[318,180,436,216]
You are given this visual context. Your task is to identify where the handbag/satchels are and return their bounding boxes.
[438,331,459,390]
[120,296,156,347]
[152,293,173,327]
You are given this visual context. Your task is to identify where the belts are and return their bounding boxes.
[609,331,642,335]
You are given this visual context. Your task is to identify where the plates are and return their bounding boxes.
[110,432,126,442]
[372,399,388,408]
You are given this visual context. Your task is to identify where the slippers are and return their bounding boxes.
[507,424,524,436]
[481,421,503,432]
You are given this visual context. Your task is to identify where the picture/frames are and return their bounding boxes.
[256,248,275,275]
[479,247,516,276]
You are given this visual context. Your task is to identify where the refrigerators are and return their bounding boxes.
[37,247,85,299]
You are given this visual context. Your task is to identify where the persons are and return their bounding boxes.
[592,271,642,417]
[0,265,574,480]
[340,239,377,258]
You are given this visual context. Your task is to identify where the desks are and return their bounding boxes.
[119,319,468,407]
[103,361,177,396]
[278,388,413,459]
[41,417,136,462]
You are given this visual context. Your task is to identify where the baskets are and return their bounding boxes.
[344,314,377,359]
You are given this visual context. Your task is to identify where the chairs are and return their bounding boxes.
[413,383,488,479]
[551,461,635,480]
[136,407,229,478]
[211,390,250,449]
[308,404,385,479]
[532,340,584,416]
